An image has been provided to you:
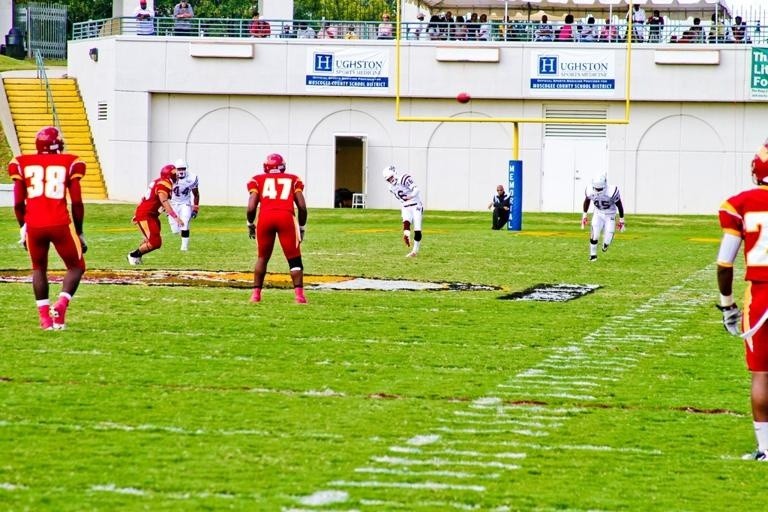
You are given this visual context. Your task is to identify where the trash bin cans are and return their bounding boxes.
[5,30,25,59]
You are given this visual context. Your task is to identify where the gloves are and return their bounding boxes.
[716,293,741,336]
[19,210,305,253]
[582,217,588,229]
[618,223,625,232]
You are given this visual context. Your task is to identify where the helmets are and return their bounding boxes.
[751,144,768,186]
[592,177,606,196]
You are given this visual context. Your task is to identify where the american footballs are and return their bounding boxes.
[456,94,471,103]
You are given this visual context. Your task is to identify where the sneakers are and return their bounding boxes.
[128,251,144,265]
[403,235,410,247]
[39,301,66,331]
[588,255,597,261]
[742,449,768,461]
[407,252,417,257]
[602,243,608,252]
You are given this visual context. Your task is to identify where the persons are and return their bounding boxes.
[578,168,625,262]
[709,134,768,468]
[133,0,768,46]
[6,126,87,330]
[245,153,307,304]
[487,184,512,230]
[165,158,199,251]
[124,164,185,265]
[381,164,422,258]
